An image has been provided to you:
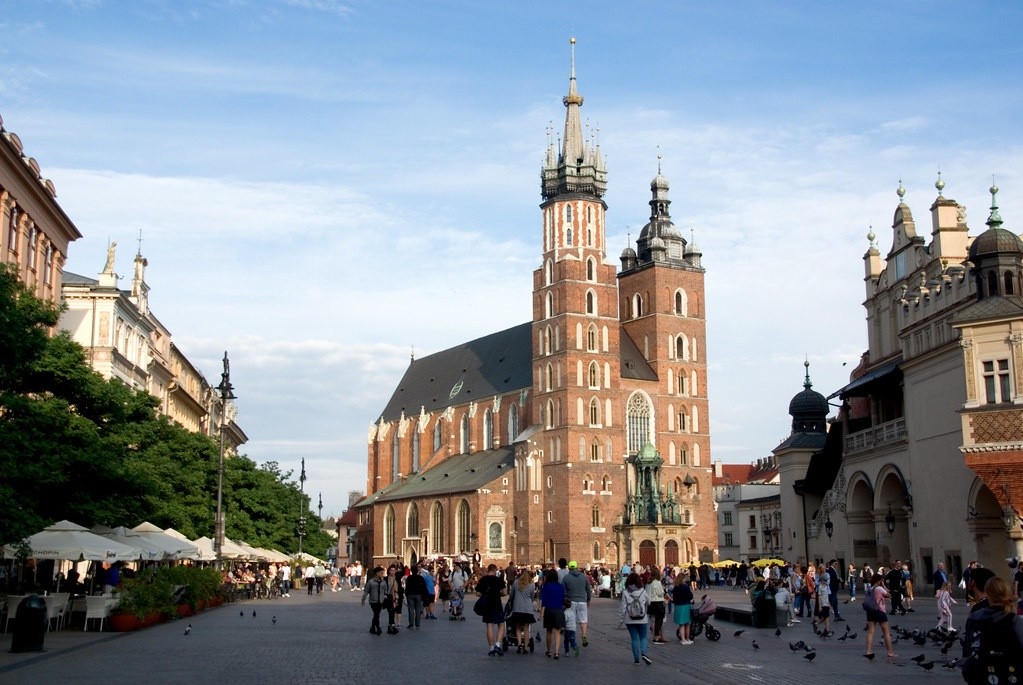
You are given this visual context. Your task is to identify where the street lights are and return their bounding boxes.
[297,456,307,562]
[211,349,238,574]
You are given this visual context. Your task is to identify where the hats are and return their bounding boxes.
[568,561,577,569]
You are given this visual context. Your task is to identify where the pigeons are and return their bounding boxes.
[862,623,869,632]
[253,609,257,619]
[845,624,852,632]
[183,623,193,636]
[841,599,849,605]
[272,615,277,624]
[788,640,817,664]
[818,627,830,640]
[774,627,782,638]
[877,624,967,673]
[733,629,746,638]
[239,610,245,619]
[534,631,541,642]
[842,362,847,367]
[751,639,761,651]
[862,652,876,662]
[836,631,848,642]
[847,632,858,641]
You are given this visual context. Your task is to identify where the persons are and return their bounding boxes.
[618,572,652,666]
[645,571,669,646]
[589,562,713,598]
[752,559,846,638]
[933,562,948,621]
[671,572,694,646]
[474,556,594,661]
[33,556,216,629]
[865,573,899,658]
[935,581,957,632]
[714,561,816,589]
[860,559,917,616]
[846,563,858,602]
[958,559,1023,685]
[357,547,486,636]
[199,551,366,600]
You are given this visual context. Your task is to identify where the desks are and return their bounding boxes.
[68,597,121,629]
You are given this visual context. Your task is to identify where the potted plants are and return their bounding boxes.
[110,563,224,633]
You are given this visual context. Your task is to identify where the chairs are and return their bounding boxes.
[4,593,129,632]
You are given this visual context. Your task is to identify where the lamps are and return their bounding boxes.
[884,479,914,539]
[764,504,782,544]
[824,494,847,541]
[988,469,1019,534]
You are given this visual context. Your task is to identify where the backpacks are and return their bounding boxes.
[861,586,883,611]
[625,589,647,619]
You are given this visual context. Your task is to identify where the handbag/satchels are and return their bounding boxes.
[474,593,489,614]
[382,594,391,609]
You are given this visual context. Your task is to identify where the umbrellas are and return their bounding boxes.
[92,517,333,571]
[4,520,136,628]
[684,558,789,576]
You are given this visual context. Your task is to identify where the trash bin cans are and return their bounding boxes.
[748,587,777,626]
[11,596,47,652]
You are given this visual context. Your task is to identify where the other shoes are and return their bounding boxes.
[565,653,570,657]
[494,646,504,656]
[488,650,495,656]
[935,625,956,632]
[813,623,818,634]
[516,645,523,654]
[651,639,667,645]
[642,655,652,665]
[280,586,363,598]
[553,653,559,659]
[681,640,693,645]
[582,635,588,648]
[574,646,580,657]
[889,608,915,616]
[634,660,639,665]
[523,649,528,654]
[369,613,437,635]
[545,651,551,657]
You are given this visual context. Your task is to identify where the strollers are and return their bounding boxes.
[676,595,721,642]
[503,594,535,653]
[448,585,466,622]
